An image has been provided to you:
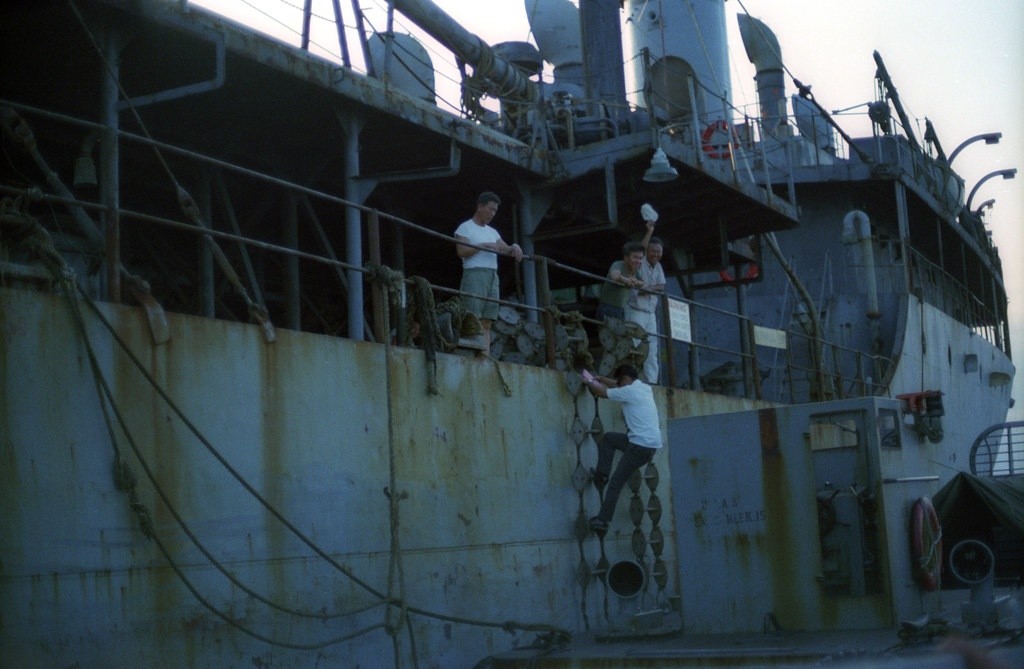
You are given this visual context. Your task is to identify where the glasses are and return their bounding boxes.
[617,375,625,387]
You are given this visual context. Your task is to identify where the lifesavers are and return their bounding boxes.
[719,260,759,287]
[702,120,738,160]
[910,497,942,591]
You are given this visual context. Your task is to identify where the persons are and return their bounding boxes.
[390,320,420,348]
[455,192,524,358]
[625,219,666,386]
[598,241,648,324]
[582,364,662,531]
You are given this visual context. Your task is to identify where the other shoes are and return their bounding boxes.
[589,515,610,532]
[591,466,609,485]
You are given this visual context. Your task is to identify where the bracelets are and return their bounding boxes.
[588,377,595,384]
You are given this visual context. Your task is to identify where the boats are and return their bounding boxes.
[0,1,1024,669]
